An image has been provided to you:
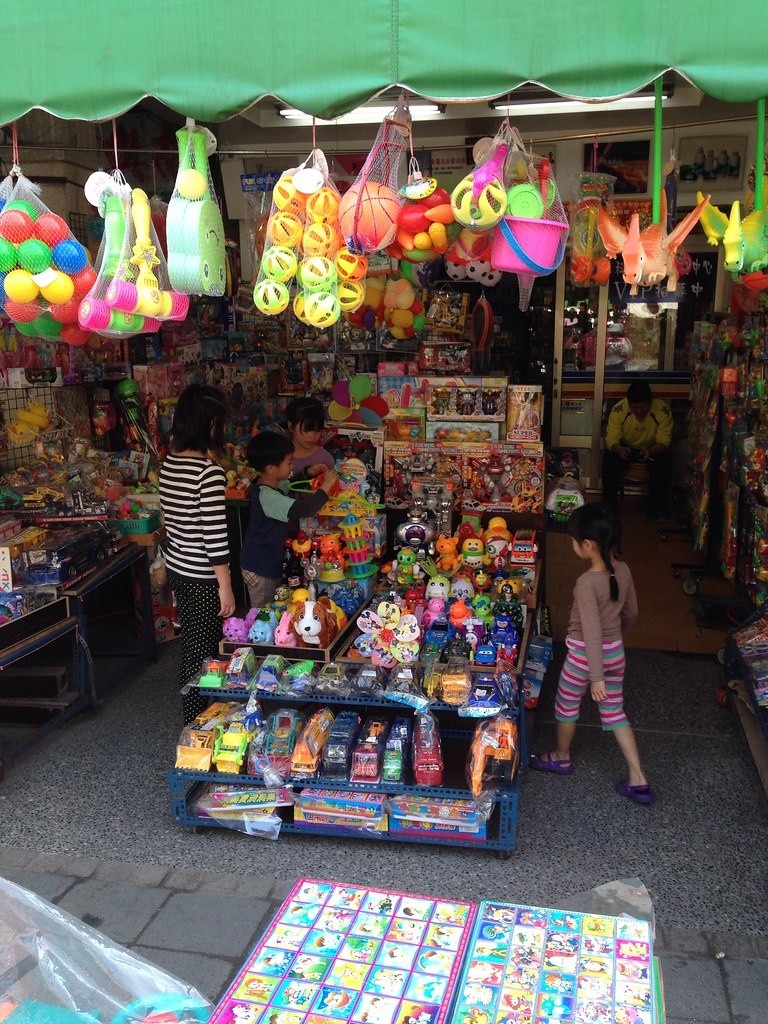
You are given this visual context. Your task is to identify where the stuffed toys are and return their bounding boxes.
[224,596,347,649]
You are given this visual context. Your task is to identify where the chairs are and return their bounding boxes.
[602,423,679,499]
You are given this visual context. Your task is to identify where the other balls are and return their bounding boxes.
[0,199,98,346]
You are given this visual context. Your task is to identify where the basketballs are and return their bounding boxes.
[339,180,401,249]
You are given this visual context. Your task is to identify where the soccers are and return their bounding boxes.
[464,260,503,286]
[445,260,466,281]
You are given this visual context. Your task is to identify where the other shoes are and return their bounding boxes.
[648,508,668,523]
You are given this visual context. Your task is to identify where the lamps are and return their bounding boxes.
[488,83,675,110]
[273,93,447,120]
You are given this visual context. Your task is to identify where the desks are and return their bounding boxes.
[0,546,157,783]
[376,508,547,606]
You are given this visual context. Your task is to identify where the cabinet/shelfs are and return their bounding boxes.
[167,661,528,861]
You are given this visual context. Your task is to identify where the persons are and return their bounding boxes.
[526,505,653,804]
[588,379,674,530]
[159,384,236,728]
[493,585,525,635]
[543,295,633,372]
[240,396,336,608]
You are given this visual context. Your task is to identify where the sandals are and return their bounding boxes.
[529,751,575,775]
[615,776,654,803]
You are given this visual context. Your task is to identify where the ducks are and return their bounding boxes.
[481,517,514,544]
[287,588,312,616]
[8,421,39,443]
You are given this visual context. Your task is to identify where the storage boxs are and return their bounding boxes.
[8,285,543,526]
[363,516,386,557]
[219,559,544,677]
[522,634,553,710]
